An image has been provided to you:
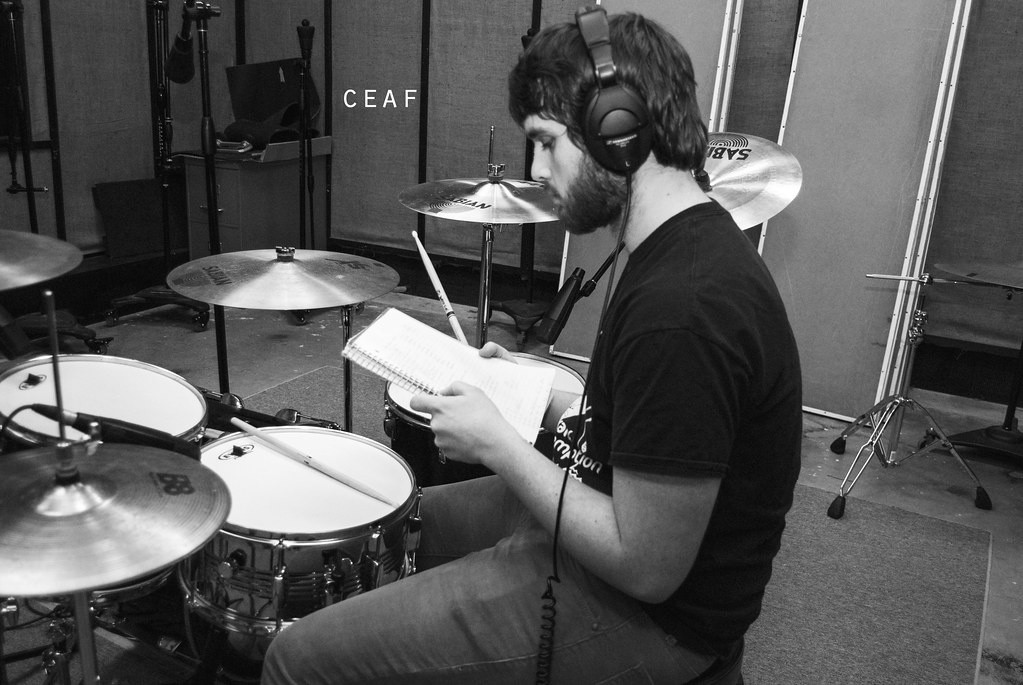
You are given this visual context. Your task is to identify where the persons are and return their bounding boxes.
[262,12,803,685]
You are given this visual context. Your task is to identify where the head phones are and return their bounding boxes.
[576,4,654,177]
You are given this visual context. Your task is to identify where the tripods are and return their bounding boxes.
[825,273,993,521]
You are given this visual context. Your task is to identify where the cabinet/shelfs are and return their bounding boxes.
[184,134,332,262]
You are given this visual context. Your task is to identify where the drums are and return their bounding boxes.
[0,351,209,606]
[383,351,587,488]
[176,424,422,638]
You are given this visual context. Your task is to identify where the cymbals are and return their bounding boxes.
[688,132,804,230]
[0,228,84,293]
[398,176,564,224]
[167,248,402,311]
[1,439,234,599]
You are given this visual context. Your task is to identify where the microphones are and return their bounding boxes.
[30,404,202,461]
[537,266,585,346]
